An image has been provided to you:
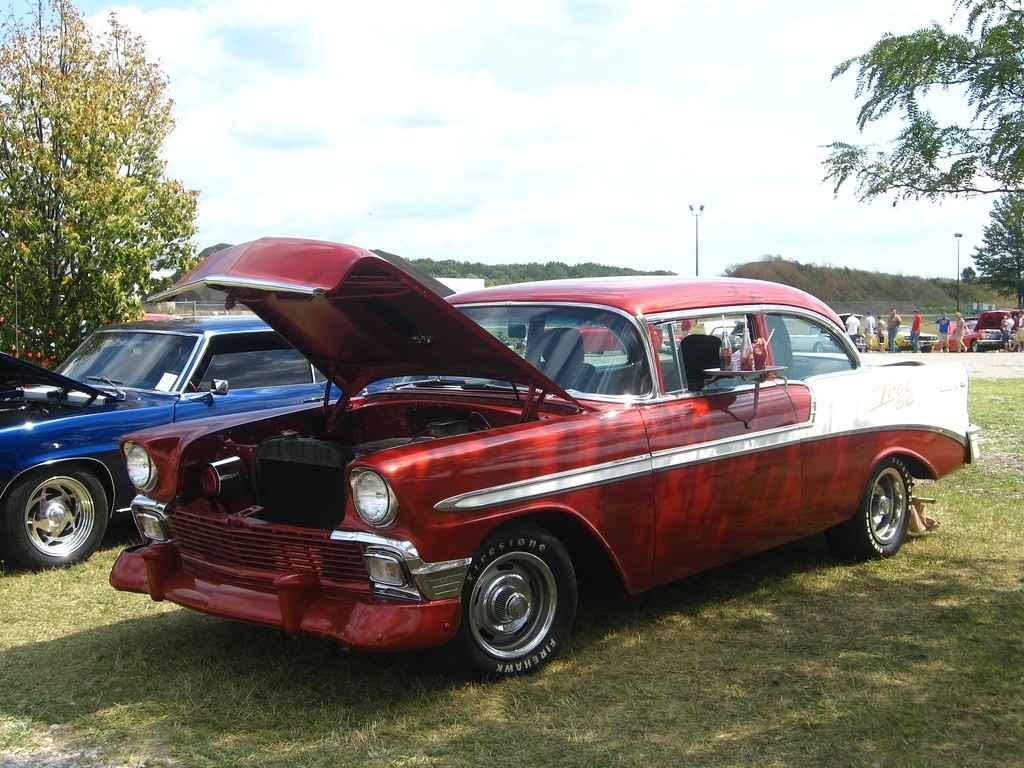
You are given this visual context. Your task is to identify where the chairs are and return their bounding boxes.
[539,326,596,393]
[661,334,722,391]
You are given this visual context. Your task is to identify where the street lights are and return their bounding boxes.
[954,231,964,313]
[689,202,706,278]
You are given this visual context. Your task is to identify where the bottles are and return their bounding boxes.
[740,328,753,371]
[719,332,732,371]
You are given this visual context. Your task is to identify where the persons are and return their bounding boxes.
[888,308,902,353]
[864,310,875,353]
[953,312,967,353]
[846,312,861,345]
[876,316,886,353]
[925,311,951,353]
[498,330,528,353]
[1001,310,1024,353]
[909,310,922,353]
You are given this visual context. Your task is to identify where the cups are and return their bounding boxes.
[753,345,768,370]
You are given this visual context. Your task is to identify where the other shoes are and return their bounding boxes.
[867,350,873,353]
[964,347,967,352]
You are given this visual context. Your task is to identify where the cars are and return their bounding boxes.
[866,323,940,353]
[934,307,1024,352]
[105,235,982,684]
[787,312,867,355]
[0,308,509,573]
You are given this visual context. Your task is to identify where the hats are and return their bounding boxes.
[913,310,919,314]
[941,311,946,315]
[866,310,872,316]
